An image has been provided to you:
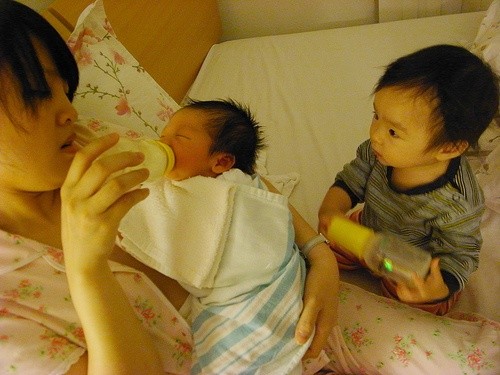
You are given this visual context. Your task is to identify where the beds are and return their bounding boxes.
[42,0,499,320]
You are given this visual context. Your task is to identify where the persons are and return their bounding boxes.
[317,44,500,318]
[0,1,500,374]
[159,95,315,374]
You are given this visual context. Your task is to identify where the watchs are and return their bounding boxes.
[300,232,330,260]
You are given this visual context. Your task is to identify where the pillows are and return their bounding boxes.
[66,0,182,139]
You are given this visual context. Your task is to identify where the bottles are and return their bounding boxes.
[326,217,432,287]
[96,138,175,184]
